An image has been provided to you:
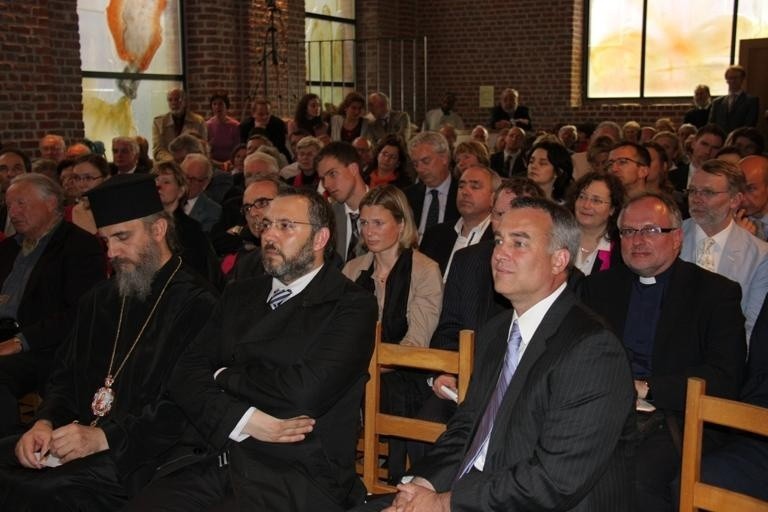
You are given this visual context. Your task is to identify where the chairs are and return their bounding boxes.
[680,379,768,512]
[357,321,479,493]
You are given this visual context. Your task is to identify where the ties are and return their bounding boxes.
[346,211,361,264]
[449,317,523,492]
[418,188,440,256]
[265,285,293,313]
[695,236,717,274]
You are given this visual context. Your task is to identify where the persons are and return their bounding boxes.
[1,86,768,510]
[707,65,759,135]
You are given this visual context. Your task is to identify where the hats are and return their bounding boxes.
[86,172,166,230]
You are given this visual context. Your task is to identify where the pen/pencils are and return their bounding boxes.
[40,418,80,462]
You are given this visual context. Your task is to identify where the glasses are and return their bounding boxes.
[239,196,275,216]
[72,174,104,182]
[257,218,325,235]
[576,193,612,206]
[606,156,645,169]
[618,224,679,239]
[684,188,731,200]
